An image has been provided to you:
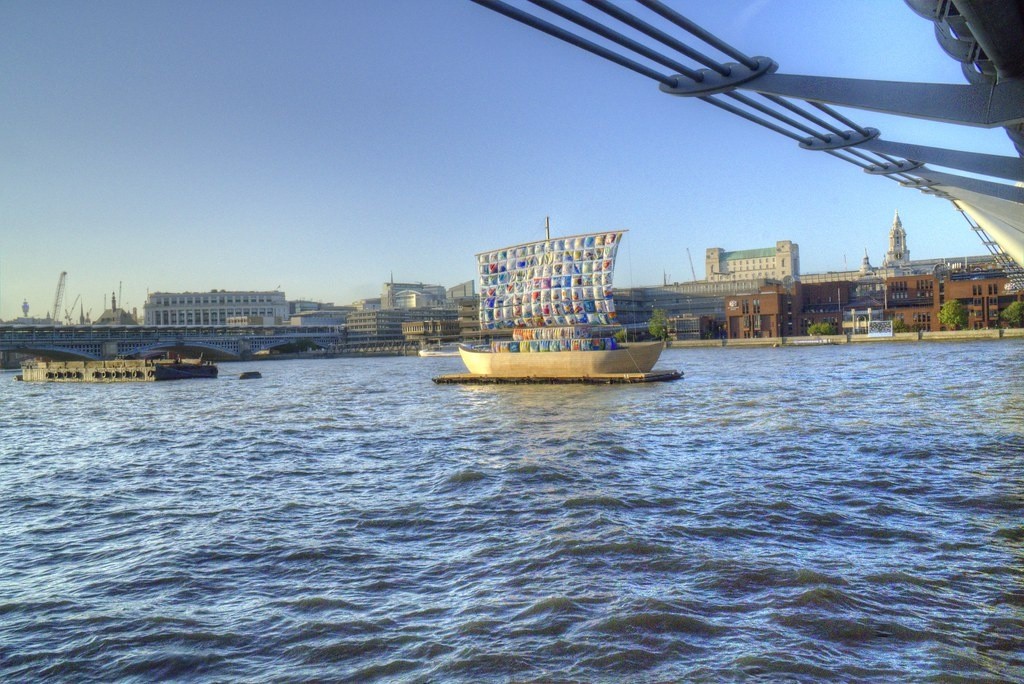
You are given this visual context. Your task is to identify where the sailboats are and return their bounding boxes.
[431,216,685,385]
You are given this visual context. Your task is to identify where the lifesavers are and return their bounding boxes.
[111,371,118,378]
[132,370,138,378]
[45,372,51,378]
[102,371,107,378]
[63,371,70,378]
[122,371,129,378]
[54,372,60,379]
[92,372,98,378]
[72,371,78,378]
[148,370,153,377]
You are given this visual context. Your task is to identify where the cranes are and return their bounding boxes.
[51,271,92,325]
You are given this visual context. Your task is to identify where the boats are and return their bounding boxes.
[417,349,461,357]
[13,352,218,381]
[239,372,262,379]
